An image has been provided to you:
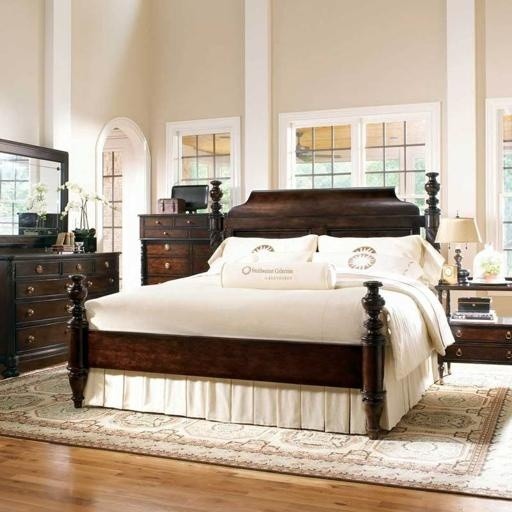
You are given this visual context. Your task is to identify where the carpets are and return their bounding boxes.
[0,361,511,501]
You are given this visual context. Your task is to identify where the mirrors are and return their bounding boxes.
[0,139,69,244]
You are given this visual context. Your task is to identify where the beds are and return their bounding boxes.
[69,171,440,440]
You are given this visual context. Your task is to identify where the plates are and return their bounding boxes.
[464,280,512,285]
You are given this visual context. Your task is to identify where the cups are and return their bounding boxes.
[75,242,84,254]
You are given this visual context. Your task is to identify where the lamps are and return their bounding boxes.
[434,216,482,284]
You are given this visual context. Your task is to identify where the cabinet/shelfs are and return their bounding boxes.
[3,249,121,378]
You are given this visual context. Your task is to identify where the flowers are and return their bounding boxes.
[56,179,118,229]
[18,182,54,236]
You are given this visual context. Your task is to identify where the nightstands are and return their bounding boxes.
[137,213,212,287]
[439,281,512,366]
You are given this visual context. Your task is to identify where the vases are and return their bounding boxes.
[72,227,97,255]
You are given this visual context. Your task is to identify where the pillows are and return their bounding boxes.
[220,257,337,290]
[318,234,425,282]
[220,234,318,276]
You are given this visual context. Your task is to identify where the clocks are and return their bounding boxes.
[442,265,458,285]
